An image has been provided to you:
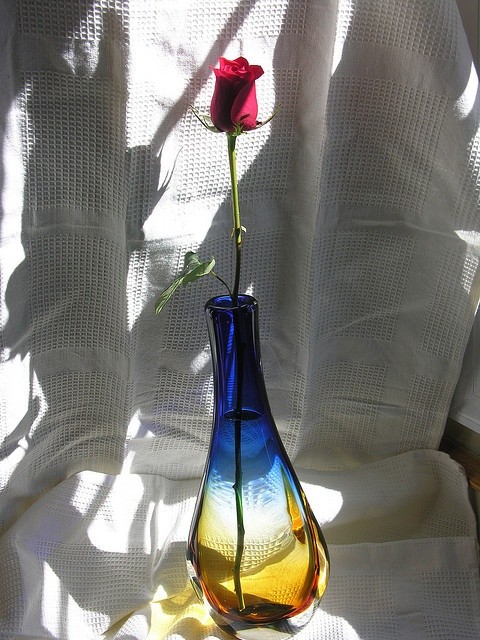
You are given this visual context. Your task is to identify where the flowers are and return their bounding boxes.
[152,56,282,611]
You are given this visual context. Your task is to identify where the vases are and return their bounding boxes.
[186,294,332,640]
